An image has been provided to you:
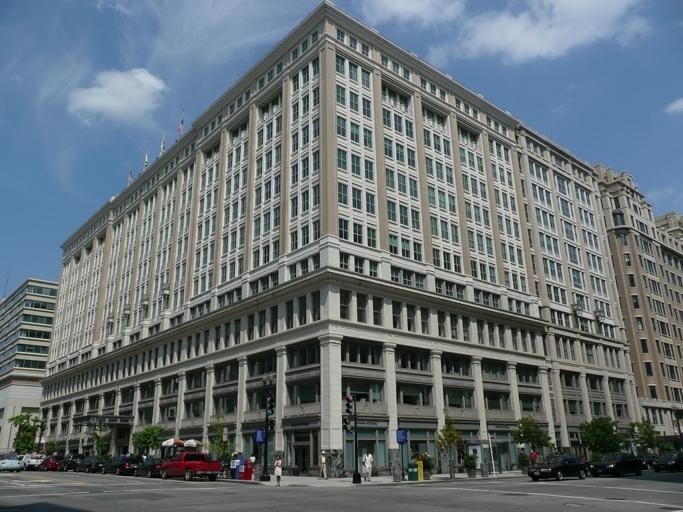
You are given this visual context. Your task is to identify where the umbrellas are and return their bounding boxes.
[161,437,184,448]
[180,438,206,448]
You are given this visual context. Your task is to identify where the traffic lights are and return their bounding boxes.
[343,417,351,432]
[345,395,353,414]
[265,397,274,416]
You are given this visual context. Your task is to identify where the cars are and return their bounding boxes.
[528,447,682,481]
[0,450,162,478]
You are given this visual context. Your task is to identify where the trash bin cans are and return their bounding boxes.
[408,463,418,481]
[422,462,432,480]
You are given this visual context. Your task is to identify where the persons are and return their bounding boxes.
[249,452,256,473]
[362,448,373,482]
[273,453,284,486]
[118,451,147,462]
[317,450,328,480]
[530,449,538,465]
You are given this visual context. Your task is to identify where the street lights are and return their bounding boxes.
[261,370,272,480]
[670,404,682,448]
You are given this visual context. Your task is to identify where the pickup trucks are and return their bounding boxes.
[158,450,221,480]
